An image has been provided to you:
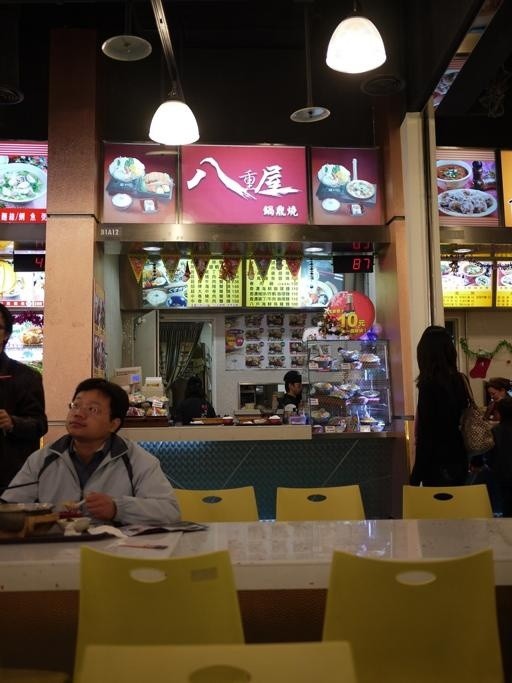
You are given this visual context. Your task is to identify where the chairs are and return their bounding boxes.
[1,480,512,682]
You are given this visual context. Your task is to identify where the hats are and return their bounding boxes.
[284,370,301,383]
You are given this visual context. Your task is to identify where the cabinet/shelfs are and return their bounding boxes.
[293,334,394,435]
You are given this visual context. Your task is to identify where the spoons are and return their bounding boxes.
[65,498,85,508]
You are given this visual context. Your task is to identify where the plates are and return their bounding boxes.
[438,188,497,216]
[461,263,485,277]
[303,278,335,306]
[110,160,146,182]
[346,179,375,199]
[13,333,43,345]
[474,275,490,286]
[441,265,452,274]
[316,163,349,188]
[501,273,512,286]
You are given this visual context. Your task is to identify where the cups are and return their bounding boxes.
[330,390,334,395]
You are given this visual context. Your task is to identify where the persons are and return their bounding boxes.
[3,377,183,525]
[0,301,50,497]
[277,370,303,409]
[484,377,512,517]
[174,376,217,425]
[409,325,472,487]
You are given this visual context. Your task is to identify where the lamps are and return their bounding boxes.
[325,0,389,77]
[141,47,200,145]
[101,0,153,64]
[288,14,331,125]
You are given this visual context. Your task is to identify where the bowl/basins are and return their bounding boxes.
[1,502,54,532]
[0,162,47,202]
[59,512,85,519]
[436,159,473,190]
[267,418,280,423]
[245,403,254,410]
[315,360,330,369]
[220,418,234,425]
[55,516,92,533]
[312,412,331,423]
[145,377,164,397]
[321,197,341,212]
[314,385,334,394]
[112,193,134,210]
[370,423,385,432]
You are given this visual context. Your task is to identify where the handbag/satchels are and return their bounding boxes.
[461,373,495,457]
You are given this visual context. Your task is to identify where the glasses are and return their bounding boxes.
[68,402,109,417]
